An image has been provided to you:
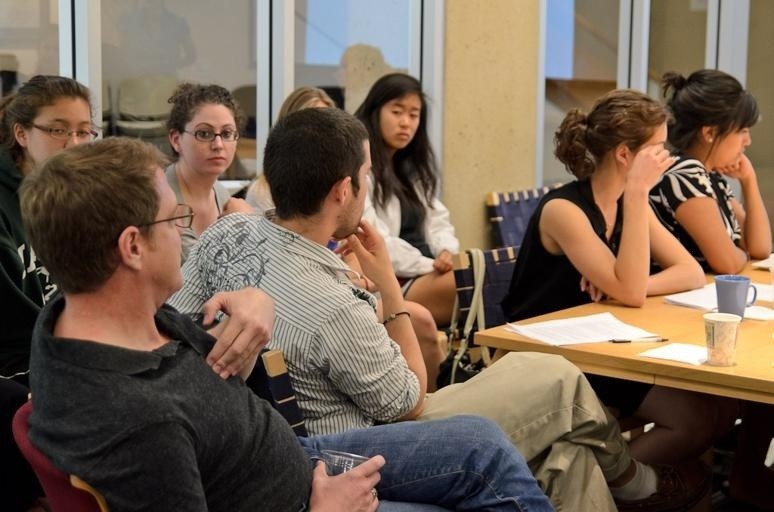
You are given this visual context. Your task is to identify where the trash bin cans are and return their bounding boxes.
[316,87,344,110]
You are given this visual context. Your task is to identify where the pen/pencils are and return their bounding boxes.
[608,338,668,343]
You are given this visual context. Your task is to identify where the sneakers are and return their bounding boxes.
[612,462,714,512]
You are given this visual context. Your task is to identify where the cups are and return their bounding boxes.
[704,312,740,366]
[321,448,370,475]
[713,274,757,319]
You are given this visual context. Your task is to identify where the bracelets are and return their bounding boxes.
[383,312,411,325]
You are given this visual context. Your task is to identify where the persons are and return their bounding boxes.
[501,87,741,468]
[246,85,377,292]
[17,135,553,512]
[0,72,95,390]
[356,72,457,325]
[164,104,713,512]
[164,80,246,272]
[648,67,773,277]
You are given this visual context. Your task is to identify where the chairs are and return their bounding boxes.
[190,314,311,438]
[11,391,111,510]
[483,183,570,261]
[450,246,654,436]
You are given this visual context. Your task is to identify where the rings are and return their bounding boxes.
[370,490,378,501]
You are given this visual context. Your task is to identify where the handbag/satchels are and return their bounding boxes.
[436,347,485,391]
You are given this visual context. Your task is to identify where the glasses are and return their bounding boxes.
[26,123,98,143]
[136,204,194,228]
[179,128,240,143]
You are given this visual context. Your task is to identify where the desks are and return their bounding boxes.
[474,247,774,409]
